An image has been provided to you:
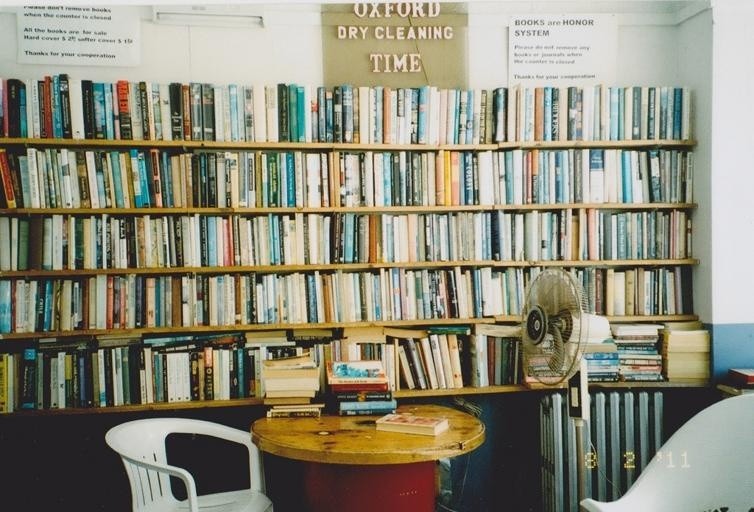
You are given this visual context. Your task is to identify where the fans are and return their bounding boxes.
[519,264,596,512]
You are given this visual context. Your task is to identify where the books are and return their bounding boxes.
[0,73,713,420]
[716,369,754,396]
[376,413,448,436]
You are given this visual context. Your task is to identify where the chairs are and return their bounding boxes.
[580,392,754,512]
[104,418,274,512]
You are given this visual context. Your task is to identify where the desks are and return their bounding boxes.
[251,400,487,512]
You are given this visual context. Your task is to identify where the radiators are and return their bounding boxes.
[538,391,663,512]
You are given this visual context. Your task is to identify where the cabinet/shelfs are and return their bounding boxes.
[0,136,697,420]
